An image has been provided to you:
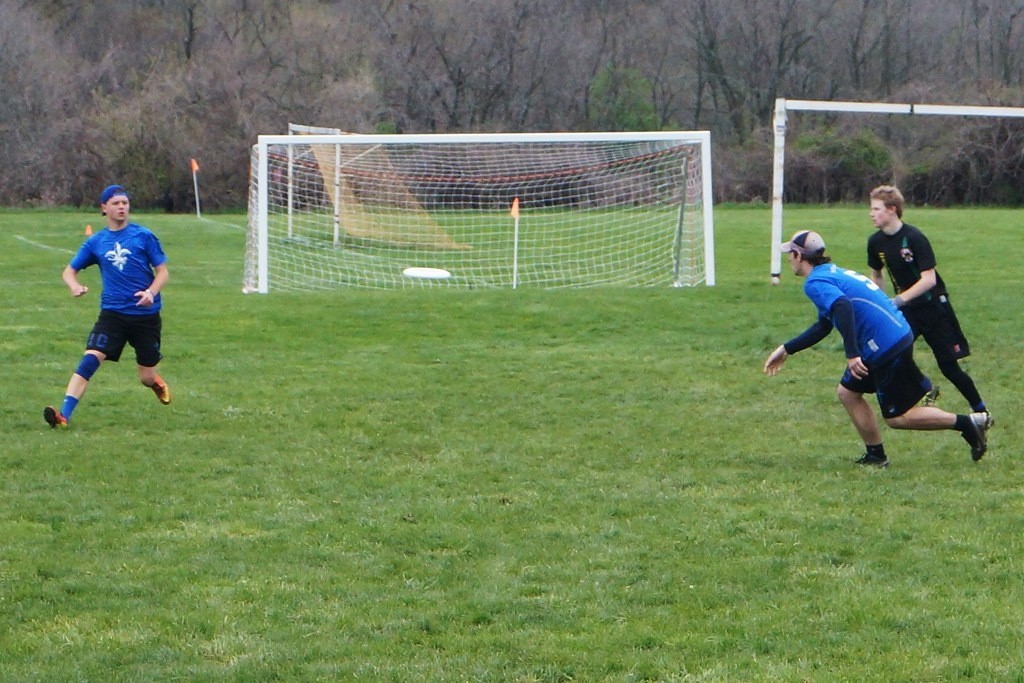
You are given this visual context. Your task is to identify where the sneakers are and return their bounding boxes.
[44,406,67,427]
[961,408,995,462]
[151,374,172,405]
[854,453,889,469]
[921,386,942,406]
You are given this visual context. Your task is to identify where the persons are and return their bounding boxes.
[866,186,993,427]
[764,229,988,468]
[44,185,171,428]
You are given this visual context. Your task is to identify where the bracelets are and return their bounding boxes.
[895,295,902,306]
[146,290,155,296]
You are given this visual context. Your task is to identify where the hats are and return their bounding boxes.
[100,185,128,216]
[779,229,826,256]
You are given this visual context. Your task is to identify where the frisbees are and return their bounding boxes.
[404,265,452,280]
